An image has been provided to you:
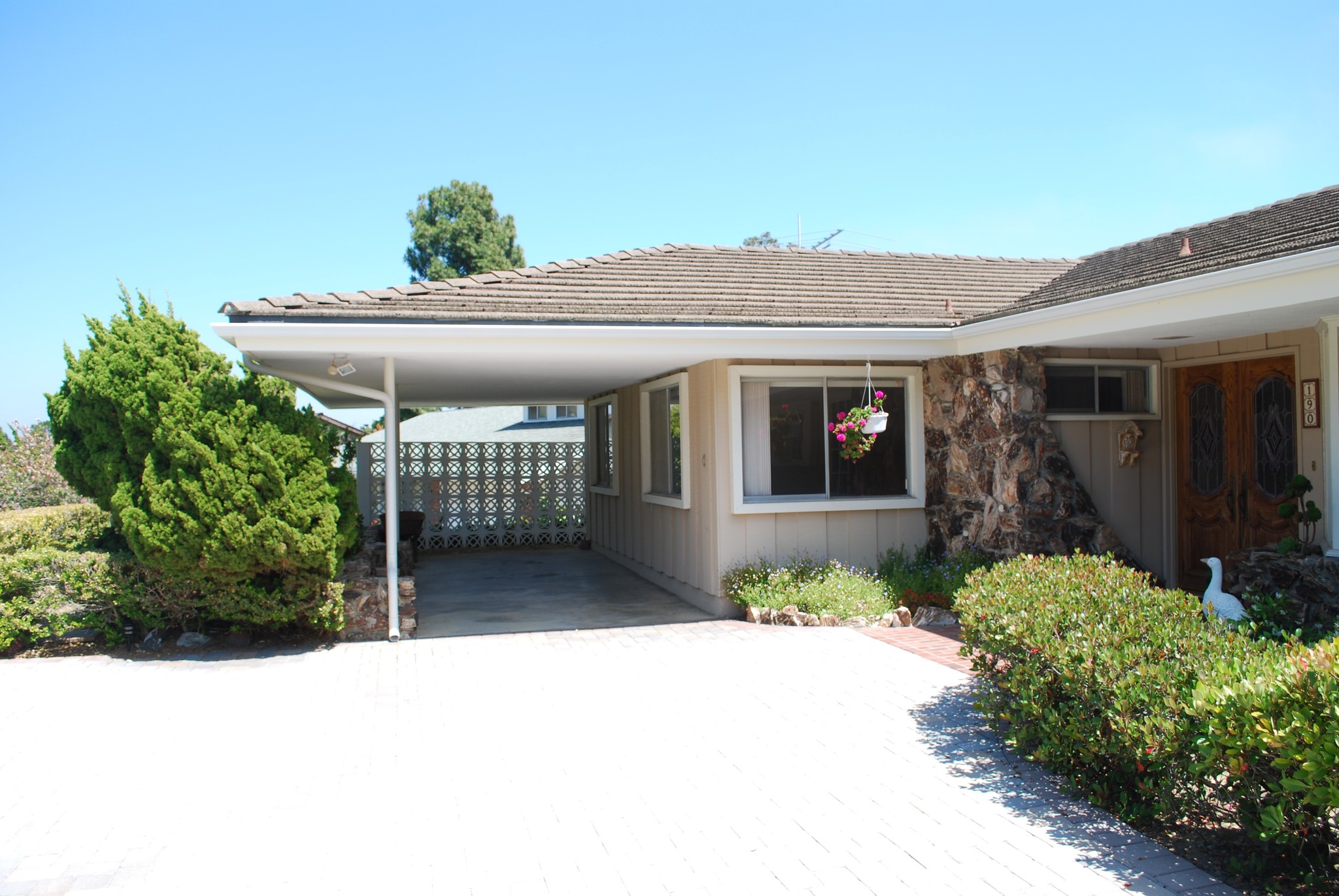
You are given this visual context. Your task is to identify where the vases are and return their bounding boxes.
[860,413,889,434]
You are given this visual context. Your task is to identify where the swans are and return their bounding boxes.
[1200,557,1252,630]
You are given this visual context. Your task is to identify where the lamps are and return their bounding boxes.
[328,356,338,375]
[336,356,356,377]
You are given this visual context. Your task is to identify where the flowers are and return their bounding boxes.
[828,390,886,464]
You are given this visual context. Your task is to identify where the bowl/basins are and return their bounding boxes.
[378,510,428,541]
